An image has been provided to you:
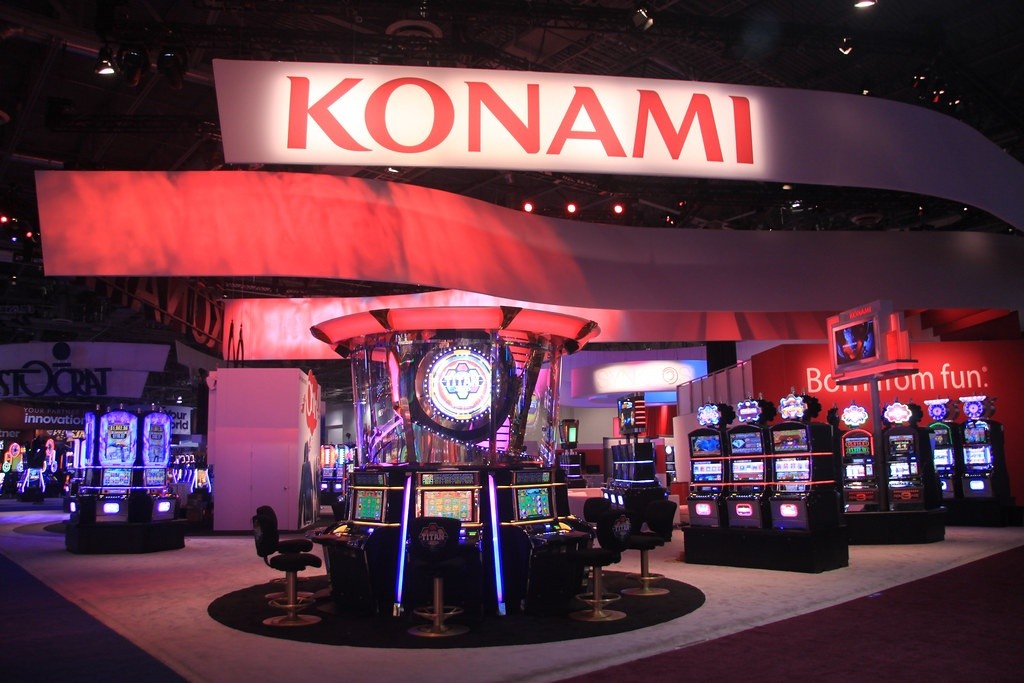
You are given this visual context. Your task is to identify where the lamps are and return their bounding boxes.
[834,23,856,58]
[94,16,149,88]
[912,61,963,107]
[628,0,657,32]
[158,24,188,89]
[661,199,692,224]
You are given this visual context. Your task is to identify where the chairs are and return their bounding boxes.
[569,488,677,621]
[252,506,323,627]
[408,517,471,639]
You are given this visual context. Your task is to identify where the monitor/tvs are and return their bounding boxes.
[845,445,993,480]
[349,487,554,523]
[100,468,168,486]
[831,311,889,374]
[690,427,809,481]
